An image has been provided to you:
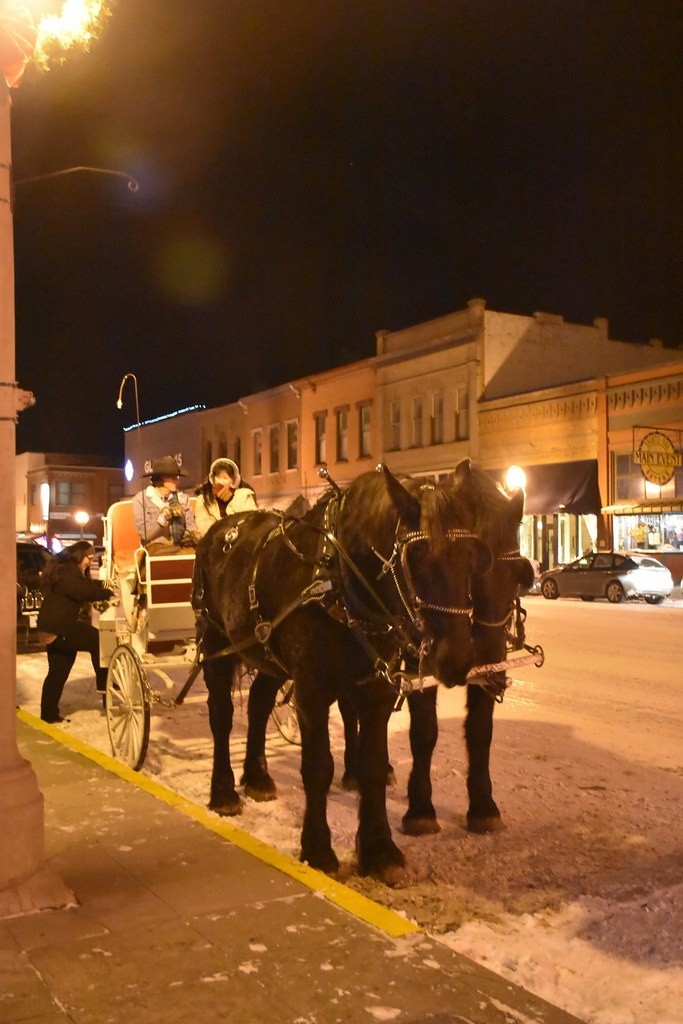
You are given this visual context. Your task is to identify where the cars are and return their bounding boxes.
[518,578,542,596]
[540,550,673,602]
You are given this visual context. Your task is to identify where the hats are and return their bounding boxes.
[140,456,188,479]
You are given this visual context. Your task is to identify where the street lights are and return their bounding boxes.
[74,509,88,542]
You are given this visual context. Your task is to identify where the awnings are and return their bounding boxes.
[480,461,603,517]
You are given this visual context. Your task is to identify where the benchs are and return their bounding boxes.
[106,496,198,620]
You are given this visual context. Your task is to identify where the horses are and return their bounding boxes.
[191,459,525,889]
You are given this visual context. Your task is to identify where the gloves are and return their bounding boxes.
[213,482,233,503]
[157,502,187,527]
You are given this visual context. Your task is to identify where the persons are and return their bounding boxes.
[37,542,124,724]
[632,523,683,549]
[134,457,256,606]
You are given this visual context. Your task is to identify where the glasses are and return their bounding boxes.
[86,556,93,563]
[160,475,180,481]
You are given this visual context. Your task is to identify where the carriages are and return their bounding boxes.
[88,456,546,891]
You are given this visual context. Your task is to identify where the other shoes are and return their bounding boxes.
[97,683,121,694]
[42,716,71,724]
